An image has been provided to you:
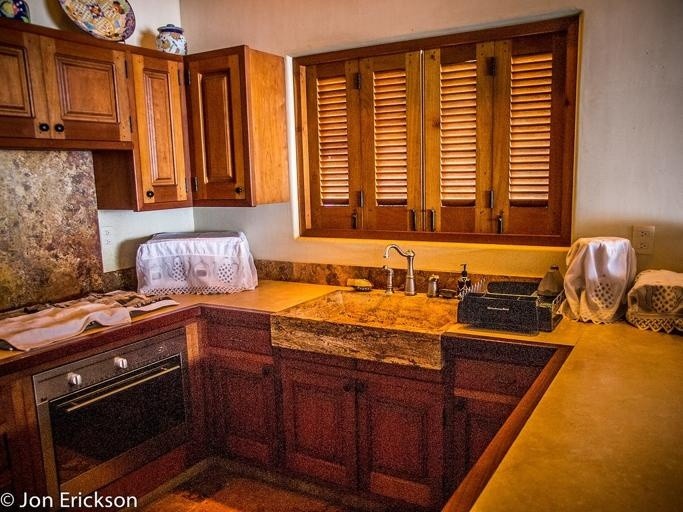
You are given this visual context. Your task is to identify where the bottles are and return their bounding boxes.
[427,273,439,298]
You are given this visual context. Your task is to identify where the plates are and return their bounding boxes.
[58,0,136,43]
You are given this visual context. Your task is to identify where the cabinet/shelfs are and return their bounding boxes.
[450,356,543,492]
[277,349,451,510]
[93,47,192,213]
[202,307,281,471]
[0,15,133,153]
[182,45,289,207]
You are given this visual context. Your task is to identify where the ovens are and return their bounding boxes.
[32,326,192,512]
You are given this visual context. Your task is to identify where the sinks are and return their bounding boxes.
[270,289,462,371]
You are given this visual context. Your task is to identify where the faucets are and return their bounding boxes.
[383,244,416,295]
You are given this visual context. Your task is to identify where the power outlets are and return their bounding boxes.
[632,225,654,255]
